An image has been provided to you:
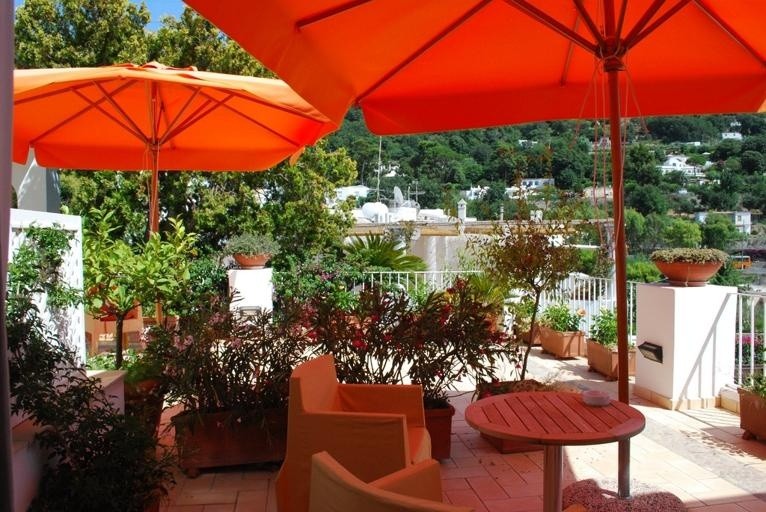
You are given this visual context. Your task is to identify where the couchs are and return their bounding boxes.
[308,449,463,510]
[275,356,432,510]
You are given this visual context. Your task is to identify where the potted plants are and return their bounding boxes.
[7,224,183,512]
[587,307,636,382]
[140,280,524,479]
[736,373,766,442]
[226,233,282,269]
[75,207,200,453]
[442,136,594,455]
[650,248,731,286]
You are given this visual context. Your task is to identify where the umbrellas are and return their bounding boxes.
[10,57,339,243]
[186,1,766,501]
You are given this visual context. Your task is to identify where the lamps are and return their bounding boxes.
[638,339,665,364]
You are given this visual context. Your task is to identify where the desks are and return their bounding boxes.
[463,391,647,512]
